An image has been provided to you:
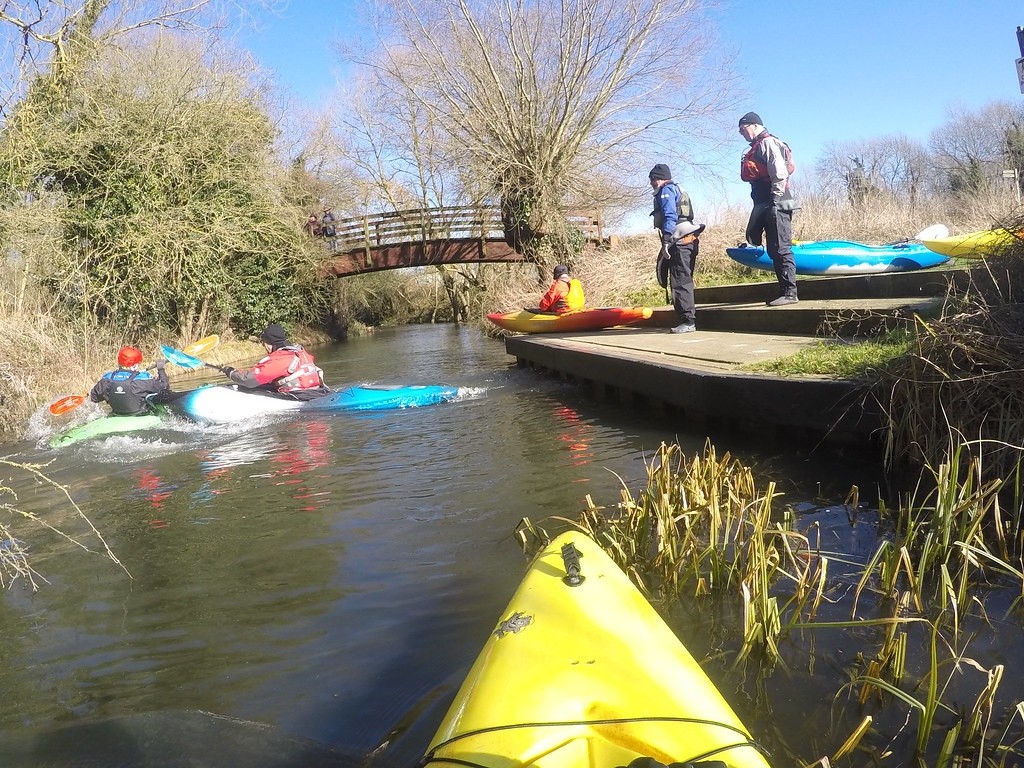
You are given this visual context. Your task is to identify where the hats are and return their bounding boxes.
[649,164,672,180]
[118,348,143,367]
[553,265,568,279]
[261,324,285,344]
[738,112,763,126]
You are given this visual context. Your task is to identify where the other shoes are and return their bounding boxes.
[671,323,696,332]
[770,294,798,306]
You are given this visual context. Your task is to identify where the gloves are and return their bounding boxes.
[223,366,234,377]
[155,359,165,370]
[767,195,781,220]
[662,244,671,259]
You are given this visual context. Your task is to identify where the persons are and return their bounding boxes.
[540,266,584,313]
[739,112,799,306]
[88,347,169,416]
[302,213,322,239]
[219,325,320,391]
[649,164,706,332]
[321,208,337,253]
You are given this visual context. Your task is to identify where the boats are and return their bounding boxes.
[726,239,953,275]
[921,225,1024,260]
[49,399,173,449]
[487,305,652,335]
[174,383,458,423]
[417,530,770,768]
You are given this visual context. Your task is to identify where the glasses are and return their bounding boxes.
[739,123,751,136]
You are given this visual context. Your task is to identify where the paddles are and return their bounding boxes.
[159,345,223,369]
[885,224,950,246]
[49,334,220,416]
